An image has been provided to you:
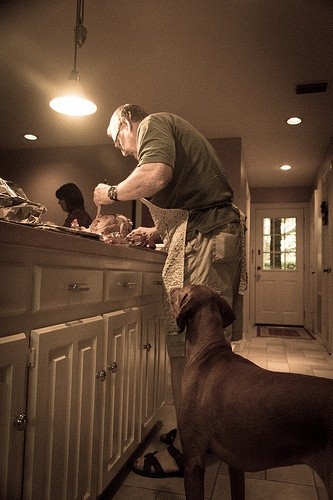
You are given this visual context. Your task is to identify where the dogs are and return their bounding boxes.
[170,284,333,500]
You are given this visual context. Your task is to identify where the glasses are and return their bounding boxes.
[114,122,122,150]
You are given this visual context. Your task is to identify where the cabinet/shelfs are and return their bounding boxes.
[1,242,30,500]
[23,246,104,500]
[97,254,144,497]
[142,262,169,440]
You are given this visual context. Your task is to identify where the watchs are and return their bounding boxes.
[108,185,120,201]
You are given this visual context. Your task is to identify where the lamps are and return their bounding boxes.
[47,0,98,117]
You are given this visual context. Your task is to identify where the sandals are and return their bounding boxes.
[160,428,177,444]
[130,436,186,478]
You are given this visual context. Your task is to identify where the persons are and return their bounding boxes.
[94,104,247,479]
[55,183,92,229]
